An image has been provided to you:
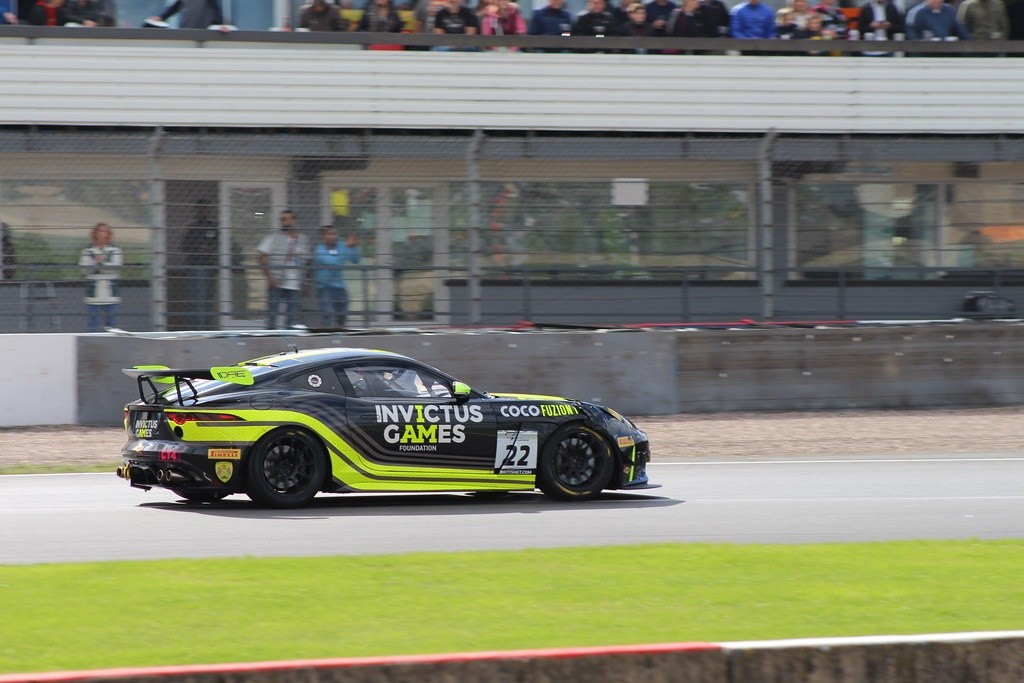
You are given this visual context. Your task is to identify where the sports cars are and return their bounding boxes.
[113,341,662,510]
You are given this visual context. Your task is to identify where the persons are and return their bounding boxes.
[0,0,116,27]
[146,0,231,33]
[181,199,244,331]
[314,225,362,329]
[281,0,1024,59]
[76,222,124,333]
[0,221,17,285]
[255,210,315,331]
[395,228,436,318]
[445,185,746,279]
